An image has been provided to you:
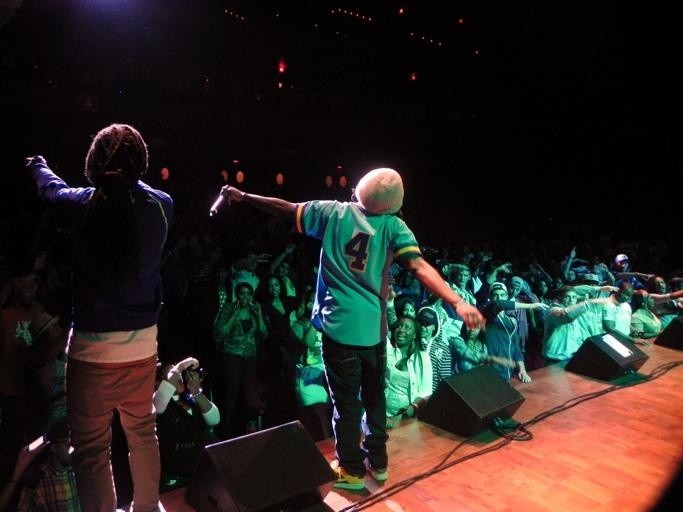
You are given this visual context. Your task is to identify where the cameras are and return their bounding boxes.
[181,368,208,392]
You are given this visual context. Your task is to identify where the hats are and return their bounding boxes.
[616,254,628,263]
[24,312,59,343]
[584,274,600,283]
[354,168,403,215]
[418,308,437,324]
[488,276,523,293]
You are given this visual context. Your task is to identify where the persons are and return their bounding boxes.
[2,193,682,512]
[18,121,171,512]
[208,163,485,493]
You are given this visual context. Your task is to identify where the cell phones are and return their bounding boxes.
[67,446,75,455]
[27,433,50,452]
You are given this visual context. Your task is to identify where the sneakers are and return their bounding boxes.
[330,458,387,490]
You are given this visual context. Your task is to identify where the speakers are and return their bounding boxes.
[416,365,524,438]
[183,420,338,511]
[653,316,683,352]
[566,330,648,379]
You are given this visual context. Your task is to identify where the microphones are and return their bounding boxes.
[207,188,231,217]
